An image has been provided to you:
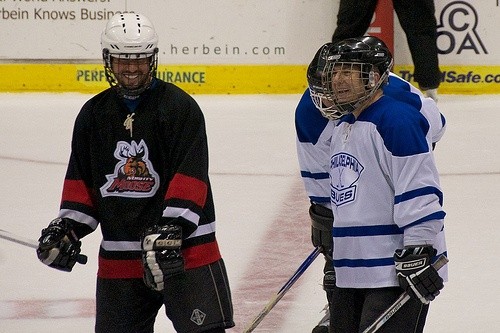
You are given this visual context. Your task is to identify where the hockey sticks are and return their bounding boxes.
[360,253,450,333]
[244,245,322,333]
[0,230,88,265]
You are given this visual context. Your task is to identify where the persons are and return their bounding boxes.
[295,0,445,333]
[37,12,235,333]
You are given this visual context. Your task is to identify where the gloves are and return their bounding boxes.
[310,204,334,262]
[394,245,443,305]
[140,223,184,293]
[36,217,82,272]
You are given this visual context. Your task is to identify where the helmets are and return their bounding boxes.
[307,43,336,94]
[328,36,393,86]
[100,13,158,72]
